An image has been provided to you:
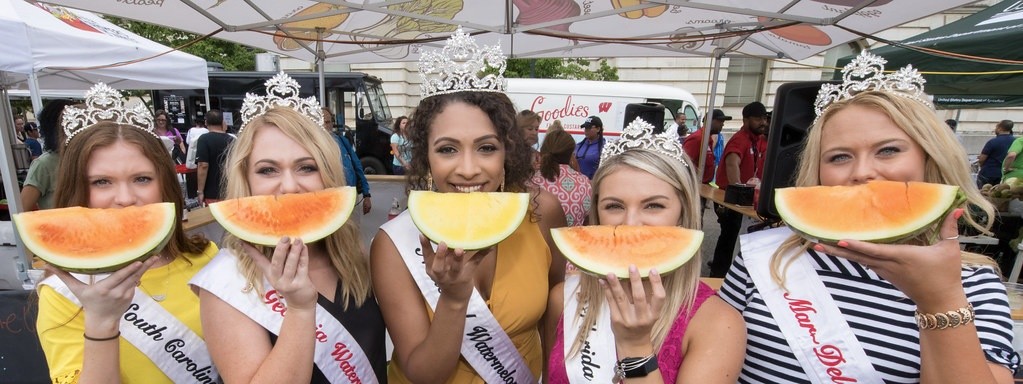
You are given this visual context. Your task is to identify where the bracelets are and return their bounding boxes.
[83,331,121,341]
[611,349,655,384]
[914,301,976,330]
[363,193,371,198]
[197,190,203,195]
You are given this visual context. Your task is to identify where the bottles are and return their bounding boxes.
[182,200,188,223]
[388,197,400,221]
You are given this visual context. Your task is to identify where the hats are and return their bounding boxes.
[25,122,37,132]
[581,116,602,128]
[743,102,771,117]
[703,110,733,120]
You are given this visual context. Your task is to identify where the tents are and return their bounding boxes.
[0,1,210,136]
[45,0,996,67]
[832,0,1023,109]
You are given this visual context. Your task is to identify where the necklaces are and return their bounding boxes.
[139,254,170,302]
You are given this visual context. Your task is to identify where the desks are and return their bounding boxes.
[700,185,783,227]
[181,207,216,232]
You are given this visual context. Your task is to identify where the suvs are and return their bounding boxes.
[148,69,430,178]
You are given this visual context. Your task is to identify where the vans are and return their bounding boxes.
[500,77,702,167]
[3,88,94,156]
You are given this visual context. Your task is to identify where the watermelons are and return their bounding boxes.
[549,225,702,280]
[208,186,356,246]
[775,181,966,246]
[12,202,178,274]
[407,190,530,251]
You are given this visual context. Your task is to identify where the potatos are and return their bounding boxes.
[979,183,1023,201]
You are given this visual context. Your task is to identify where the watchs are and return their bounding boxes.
[616,354,659,378]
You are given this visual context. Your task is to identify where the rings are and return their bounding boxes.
[135,280,141,287]
[865,263,870,269]
[945,234,960,240]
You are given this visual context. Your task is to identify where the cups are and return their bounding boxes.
[754,183,760,210]
[746,177,760,187]
[12,144,32,170]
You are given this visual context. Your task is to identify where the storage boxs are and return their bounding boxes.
[984,197,1013,212]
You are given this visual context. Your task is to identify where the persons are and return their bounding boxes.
[675,107,732,230]
[186,68,390,384]
[366,24,569,384]
[710,101,774,277]
[320,105,371,216]
[515,106,580,176]
[539,115,747,384]
[389,115,415,174]
[152,107,237,209]
[35,80,220,384]
[716,45,1021,384]
[13,98,79,213]
[532,127,595,229]
[575,114,608,181]
[945,120,965,146]
[976,119,1023,198]
